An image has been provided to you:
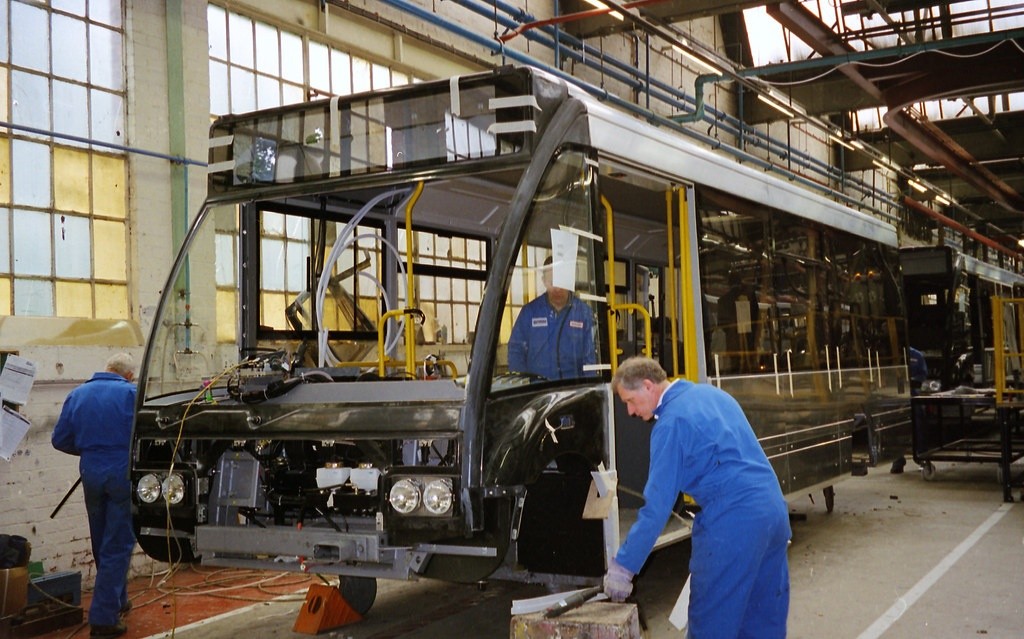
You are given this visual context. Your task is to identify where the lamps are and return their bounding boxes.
[872,154,902,173]
[672,36,736,77]
[757,85,806,119]
[584,0,640,21]
[907,179,951,207]
[828,128,865,151]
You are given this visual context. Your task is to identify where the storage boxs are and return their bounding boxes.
[0,566,29,620]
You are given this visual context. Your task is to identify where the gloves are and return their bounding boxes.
[602,557,635,603]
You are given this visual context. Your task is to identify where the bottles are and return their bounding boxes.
[204,377,212,404]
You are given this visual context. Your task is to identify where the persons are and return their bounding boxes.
[604,357,791,639]
[507,256,596,381]
[52,353,137,637]
[890,344,930,474]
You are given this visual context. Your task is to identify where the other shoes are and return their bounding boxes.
[119,600,131,612]
[90,623,127,638]
